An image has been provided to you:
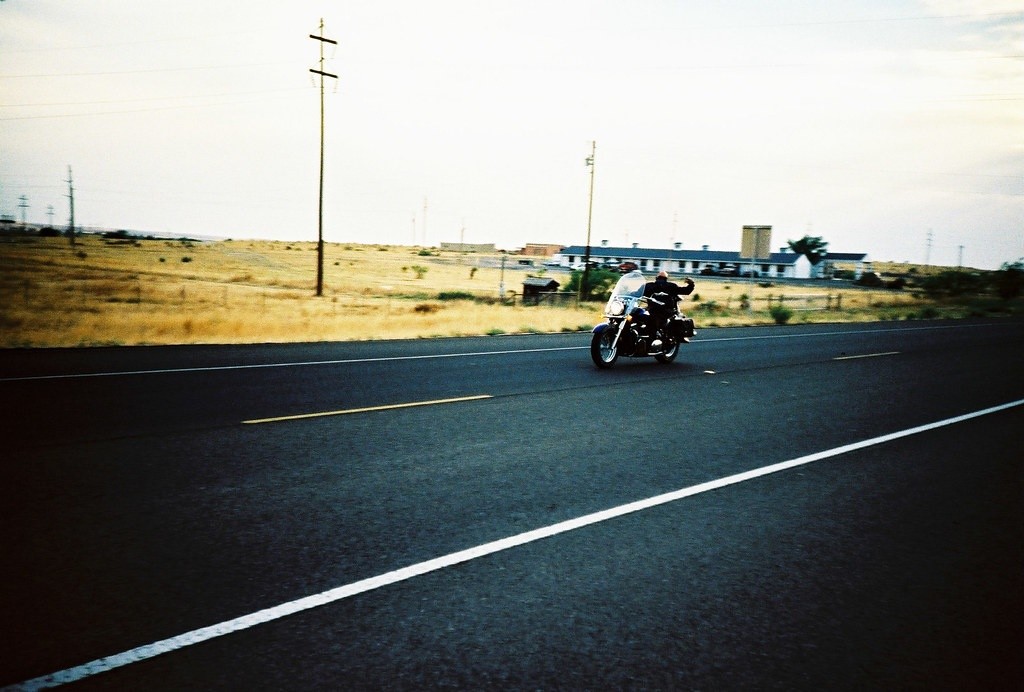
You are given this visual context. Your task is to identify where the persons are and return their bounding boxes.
[627,270,694,351]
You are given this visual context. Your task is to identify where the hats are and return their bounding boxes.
[657,271,667,278]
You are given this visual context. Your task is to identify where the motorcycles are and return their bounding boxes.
[590,269,698,370]
